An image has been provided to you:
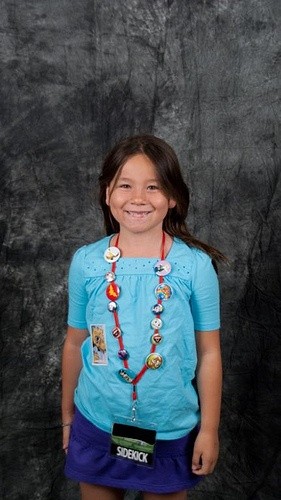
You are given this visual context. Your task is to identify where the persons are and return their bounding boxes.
[61,136,223,500]
